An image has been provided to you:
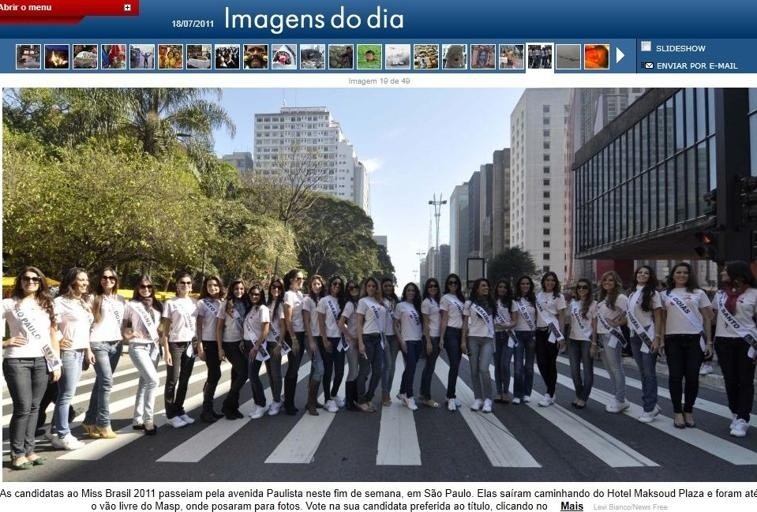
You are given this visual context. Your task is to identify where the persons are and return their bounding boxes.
[216,47,239,68]
[585,46,608,69]
[244,45,267,68]
[140,52,152,68]
[366,50,376,62]
[504,48,515,69]
[2,260,756,471]
[528,45,552,69]
[188,48,211,63]
[341,47,353,62]
[159,45,182,68]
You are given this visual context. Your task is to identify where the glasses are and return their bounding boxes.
[428,284,437,288]
[103,276,114,280]
[141,284,151,289]
[449,280,457,285]
[22,276,39,282]
[578,285,588,289]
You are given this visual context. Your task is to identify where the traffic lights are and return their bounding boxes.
[733,175,756,235]
[692,228,718,260]
[702,186,719,229]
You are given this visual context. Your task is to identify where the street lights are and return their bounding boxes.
[426,191,449,280]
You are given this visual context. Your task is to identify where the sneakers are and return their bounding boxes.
[472,395,695,428]
[305,380,461,416]
[132,401,298,435]
[730,414,750,437]
[11,422,116,469]
